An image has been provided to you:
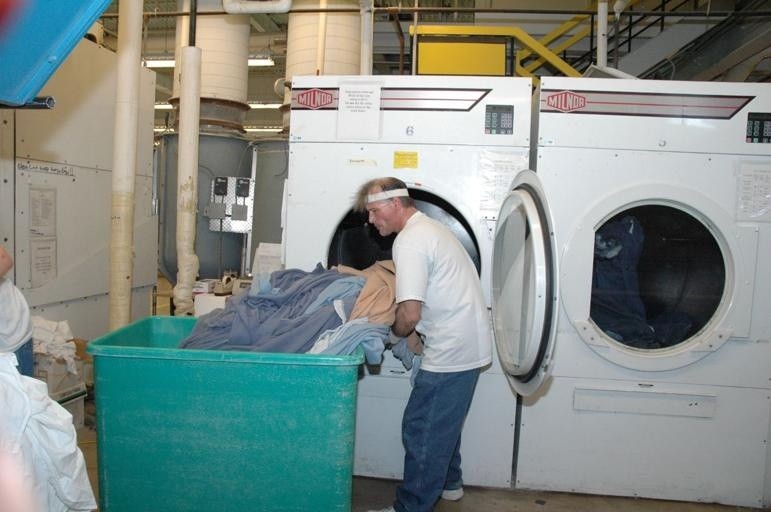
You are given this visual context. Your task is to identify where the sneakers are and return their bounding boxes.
[440,486,466,501]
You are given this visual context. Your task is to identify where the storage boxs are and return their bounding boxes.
[87,314,365,512]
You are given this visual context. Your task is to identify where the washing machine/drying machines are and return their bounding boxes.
[489,75,771,506]
[281,74,561,491]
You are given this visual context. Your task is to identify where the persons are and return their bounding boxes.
[353,176,495,512]
[1,242,35,377]
